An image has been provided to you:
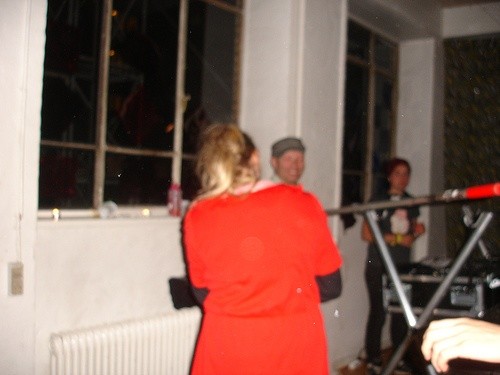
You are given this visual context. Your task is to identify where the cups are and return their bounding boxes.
[98,200,118,218]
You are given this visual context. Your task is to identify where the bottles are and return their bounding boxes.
[168,184,182,215]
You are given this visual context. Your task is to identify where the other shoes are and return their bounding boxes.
[399,363,412,371]
[371,357,382,365]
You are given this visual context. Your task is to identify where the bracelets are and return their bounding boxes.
[412,231,419,239]
[390,233,402,248]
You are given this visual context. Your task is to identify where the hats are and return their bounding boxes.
[272,139,304,157]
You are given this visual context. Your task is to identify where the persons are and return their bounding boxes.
[169,124,343,375]
[359,158,428,375]
[421,316,500,373]
[270,136,307,186]
